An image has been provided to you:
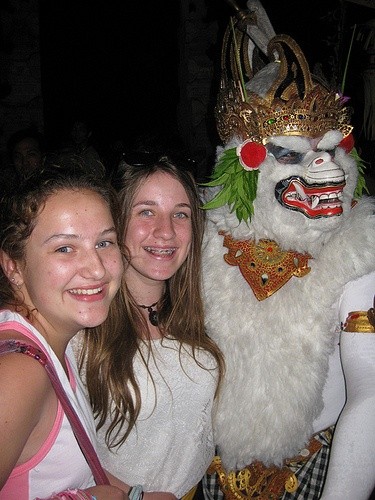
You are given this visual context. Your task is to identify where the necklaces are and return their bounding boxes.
[129,296,166,324]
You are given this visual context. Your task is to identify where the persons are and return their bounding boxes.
[0,157,125,500]
[73,151,226,500]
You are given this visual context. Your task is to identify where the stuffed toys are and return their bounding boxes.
[192,130,375,500]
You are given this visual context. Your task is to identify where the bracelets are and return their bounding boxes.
[55,488,96,500]
[128,482,144,499]
